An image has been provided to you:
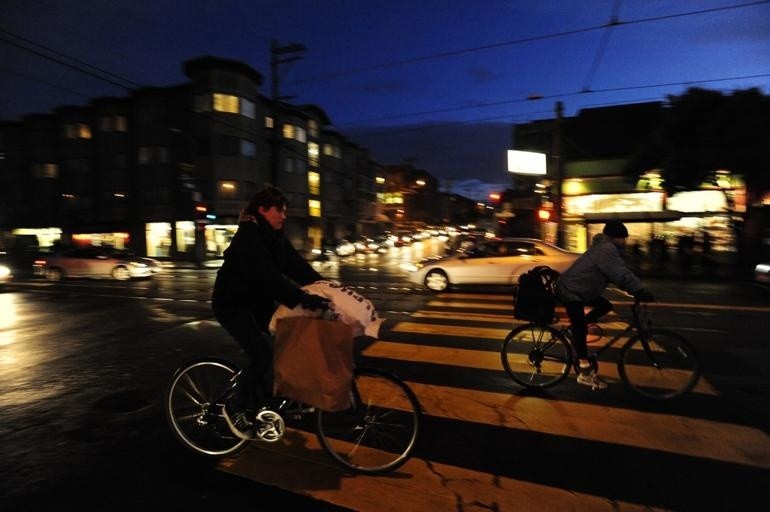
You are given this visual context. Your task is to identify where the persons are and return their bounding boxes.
[212,187,332,439]
[555,218,657,391]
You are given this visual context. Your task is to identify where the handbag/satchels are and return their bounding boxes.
[273,317,354,413]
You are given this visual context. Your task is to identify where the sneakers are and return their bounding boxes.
[576,365,608,389]
[223,403,254,441]
[512,265,560,323]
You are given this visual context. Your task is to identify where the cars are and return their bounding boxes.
[32,245,163,283]
[414,236,582,296]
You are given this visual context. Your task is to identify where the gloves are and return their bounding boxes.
[299,293,331,312]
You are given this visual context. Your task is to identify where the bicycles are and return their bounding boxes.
[164,299,425,475]
[498,293,706,403]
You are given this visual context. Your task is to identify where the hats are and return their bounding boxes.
[603,218,628,238]
